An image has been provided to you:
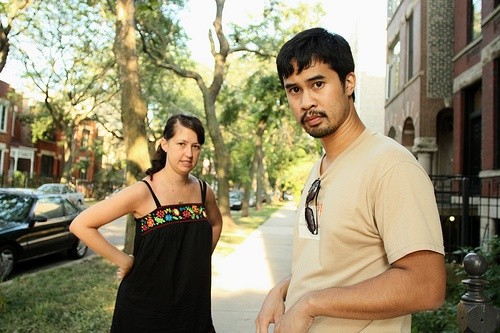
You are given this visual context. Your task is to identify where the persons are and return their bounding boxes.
[69,115,224,333]
[255,28,447,333]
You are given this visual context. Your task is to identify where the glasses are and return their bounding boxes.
[305,177,321,235]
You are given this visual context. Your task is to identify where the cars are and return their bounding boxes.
[37,183,85,207]
[0,187,91,284]
[229,189,267,209]
